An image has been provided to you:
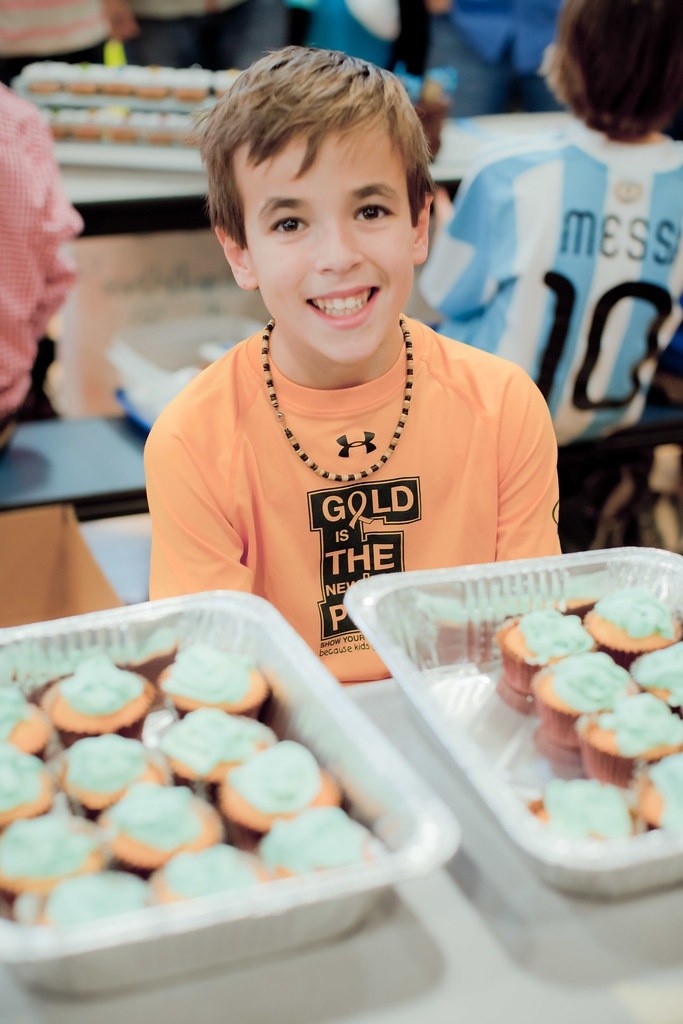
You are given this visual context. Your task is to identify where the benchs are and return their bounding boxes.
[0,392,683,524]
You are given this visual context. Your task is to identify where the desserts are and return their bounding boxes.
[498,588,683,842]
[168,340,235,382]
[17,63,246,140]
[0,638,388,923]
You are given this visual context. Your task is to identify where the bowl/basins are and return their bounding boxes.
[0,590,460,995]
[109,316,268,432]
[343,546,683,897]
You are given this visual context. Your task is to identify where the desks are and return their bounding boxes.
[54,107,594,239]
[0,675,683,1024]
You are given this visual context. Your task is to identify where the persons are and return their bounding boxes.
[143,48,563,687]
[419,0,683,447]
[0,82,85,416]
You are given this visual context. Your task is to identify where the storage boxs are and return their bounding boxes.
[0,589,461,1000]
[347,541,683,900]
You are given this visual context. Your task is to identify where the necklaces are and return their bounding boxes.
[261,318,414,482]
